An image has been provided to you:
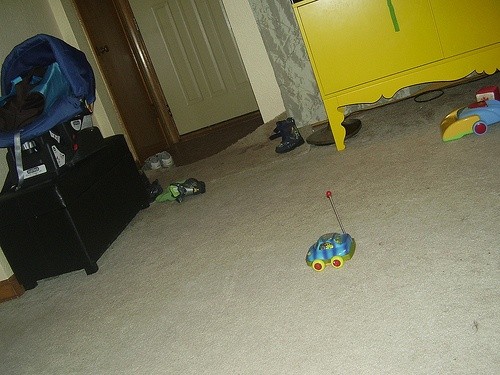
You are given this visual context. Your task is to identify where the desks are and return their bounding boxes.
[0,134,150,290]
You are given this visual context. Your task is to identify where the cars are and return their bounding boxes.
[304,233,355,271]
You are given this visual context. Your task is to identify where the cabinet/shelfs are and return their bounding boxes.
[292,0,500,151]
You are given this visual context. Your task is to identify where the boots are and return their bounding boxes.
[275,123,305,154]
[169,176,206,202]
[143,179,163,200]
[268,118,287,141]
